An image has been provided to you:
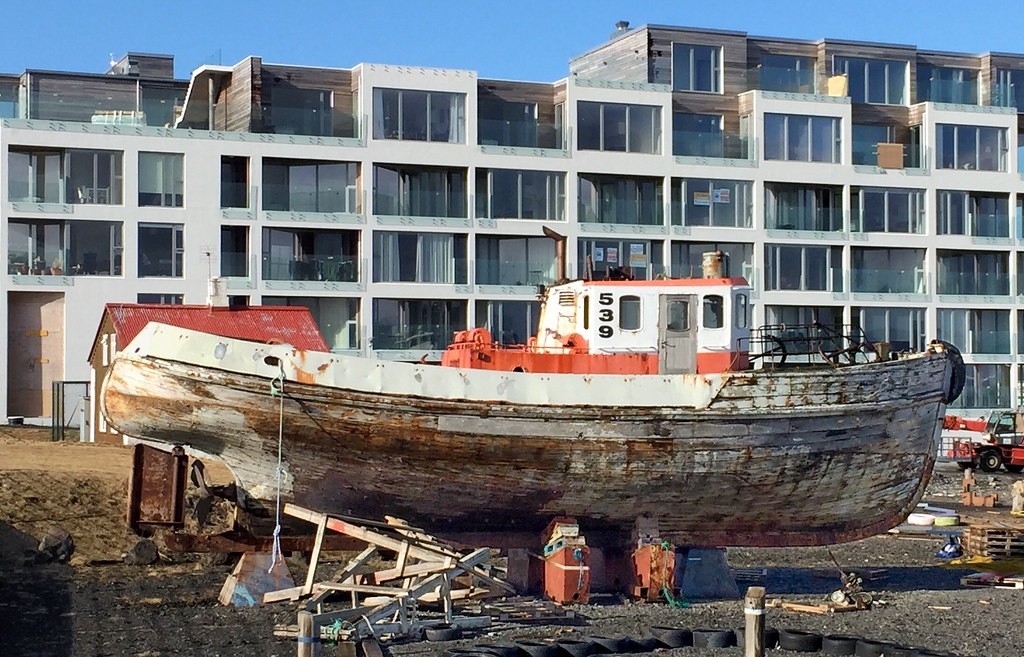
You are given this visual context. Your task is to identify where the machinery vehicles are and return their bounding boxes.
[937,408,1024,473]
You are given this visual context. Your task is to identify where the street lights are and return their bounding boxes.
[203,250,210,311]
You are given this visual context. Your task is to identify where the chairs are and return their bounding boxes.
[77,185,92,204]
[288,256,353,280]
[74,252,98,275]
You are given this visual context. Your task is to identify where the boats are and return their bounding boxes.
[86,226,966,552]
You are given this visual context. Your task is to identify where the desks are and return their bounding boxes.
[87,188,111,205]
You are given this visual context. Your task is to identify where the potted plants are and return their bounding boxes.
[20,252,63,275]
